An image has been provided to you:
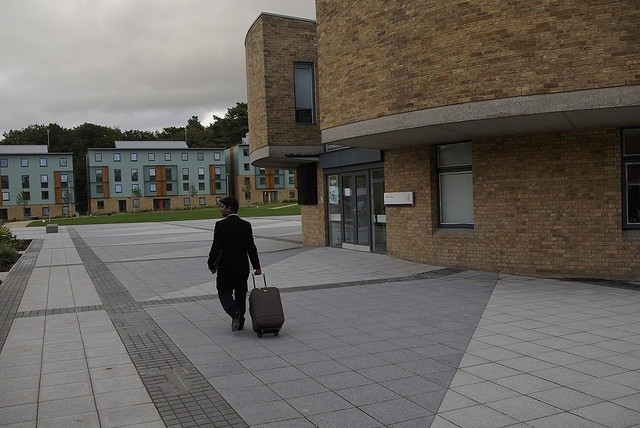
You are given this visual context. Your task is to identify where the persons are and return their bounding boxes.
[207,197,263,333]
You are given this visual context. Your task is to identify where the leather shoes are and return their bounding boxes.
[232,311,241,331]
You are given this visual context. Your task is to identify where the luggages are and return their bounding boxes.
[249,273,284,338]
